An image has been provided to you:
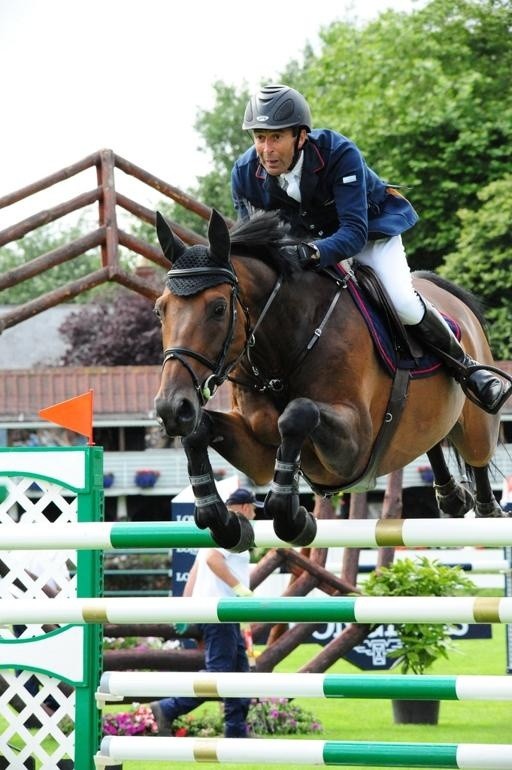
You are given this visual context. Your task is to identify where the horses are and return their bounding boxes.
[152,207,512,555]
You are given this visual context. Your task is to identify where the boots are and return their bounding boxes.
[403,289,505,410]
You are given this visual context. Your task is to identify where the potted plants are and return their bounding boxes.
[346,555,475,724]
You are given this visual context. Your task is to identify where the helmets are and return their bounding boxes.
[242,85,313,132]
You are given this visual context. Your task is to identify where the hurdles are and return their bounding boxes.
[1,448,512,770]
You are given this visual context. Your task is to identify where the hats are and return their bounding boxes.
[226,489,264,509]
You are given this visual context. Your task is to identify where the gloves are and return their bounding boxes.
[281,243,317,268]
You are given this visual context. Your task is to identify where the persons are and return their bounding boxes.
[229,83,507,414]
[147,488,264,738]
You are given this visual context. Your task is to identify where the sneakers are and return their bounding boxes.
[150,701,174,737]
